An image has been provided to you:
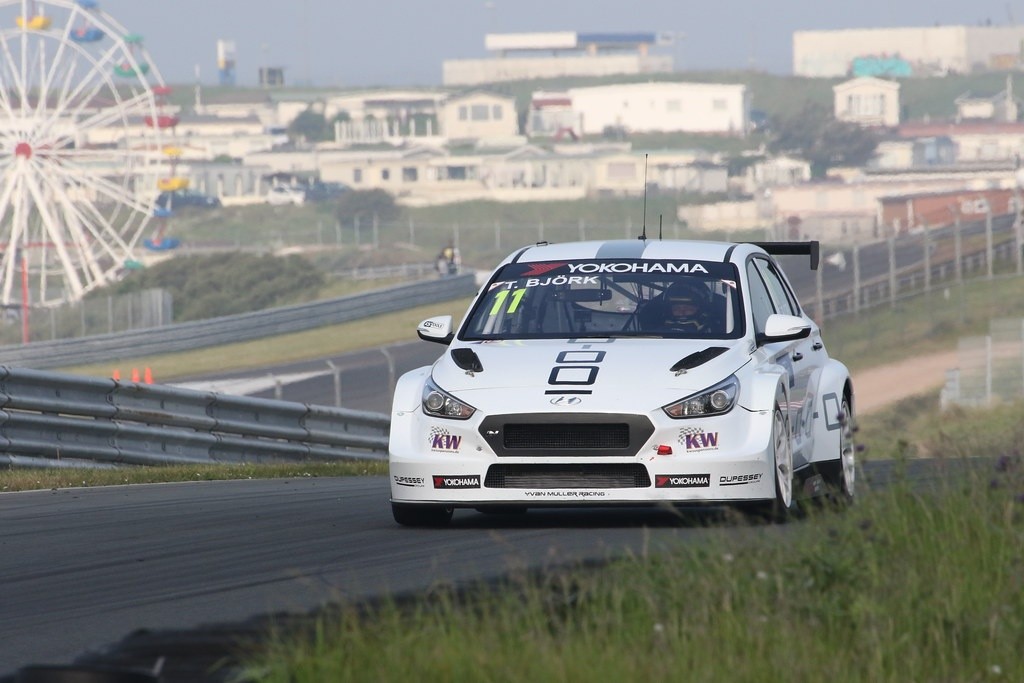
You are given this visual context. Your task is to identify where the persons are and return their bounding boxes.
[652,277,726,335]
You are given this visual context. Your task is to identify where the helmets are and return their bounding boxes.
[662,278,713,334]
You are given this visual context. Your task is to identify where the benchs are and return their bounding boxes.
[537,296,640,332]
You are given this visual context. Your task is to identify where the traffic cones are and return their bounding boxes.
[113,369,121,380]
[131,367,141,383]
[144,367,156,385]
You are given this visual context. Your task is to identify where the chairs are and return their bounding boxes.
[636,292,727,332]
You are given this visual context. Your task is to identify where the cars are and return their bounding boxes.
[157,188,222,211]
[386,237,859,527]
